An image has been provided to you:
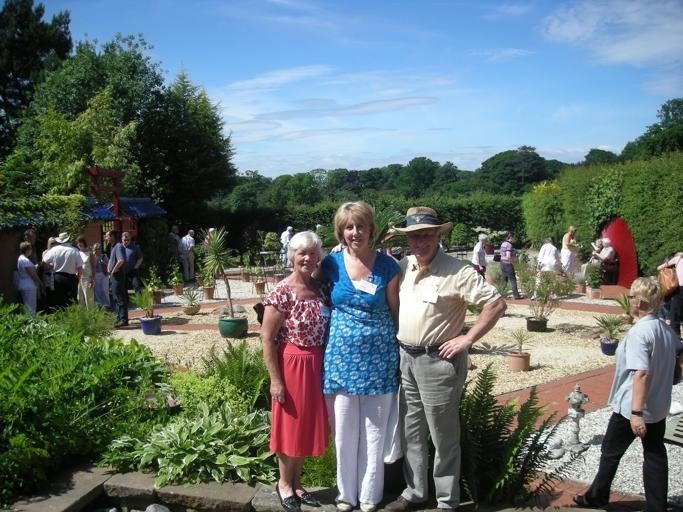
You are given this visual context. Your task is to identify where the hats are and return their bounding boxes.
[390,204,455,238]
[51,229,75,247]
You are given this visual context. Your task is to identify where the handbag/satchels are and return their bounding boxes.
[493,254,501,262]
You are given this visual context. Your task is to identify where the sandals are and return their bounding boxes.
[571,491,609,508]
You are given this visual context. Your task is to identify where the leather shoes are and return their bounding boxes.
[275,482,319,508]
[335,499,377,512]
[380,493,430,512]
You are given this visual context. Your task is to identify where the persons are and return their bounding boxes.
[571,275,682,512]
[15,226,217,328]
[260,230,333,512]
[655,251,683,341]
[329,206,508,512]
[312,201,402,512]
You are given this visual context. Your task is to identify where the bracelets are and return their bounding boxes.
[631,410,644,417]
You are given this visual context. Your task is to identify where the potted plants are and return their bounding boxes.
[128,226,287,338]
[467,260,634,372]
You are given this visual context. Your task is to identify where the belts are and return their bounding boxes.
[398,340,441,354]
